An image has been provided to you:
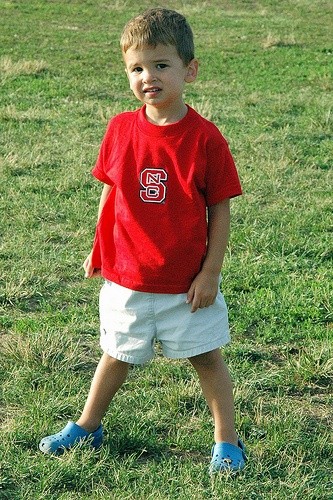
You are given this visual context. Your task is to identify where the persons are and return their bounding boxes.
[40,8,255,479]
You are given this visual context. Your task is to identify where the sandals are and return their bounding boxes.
[209,440,248,475]
[39,421,103,455]
